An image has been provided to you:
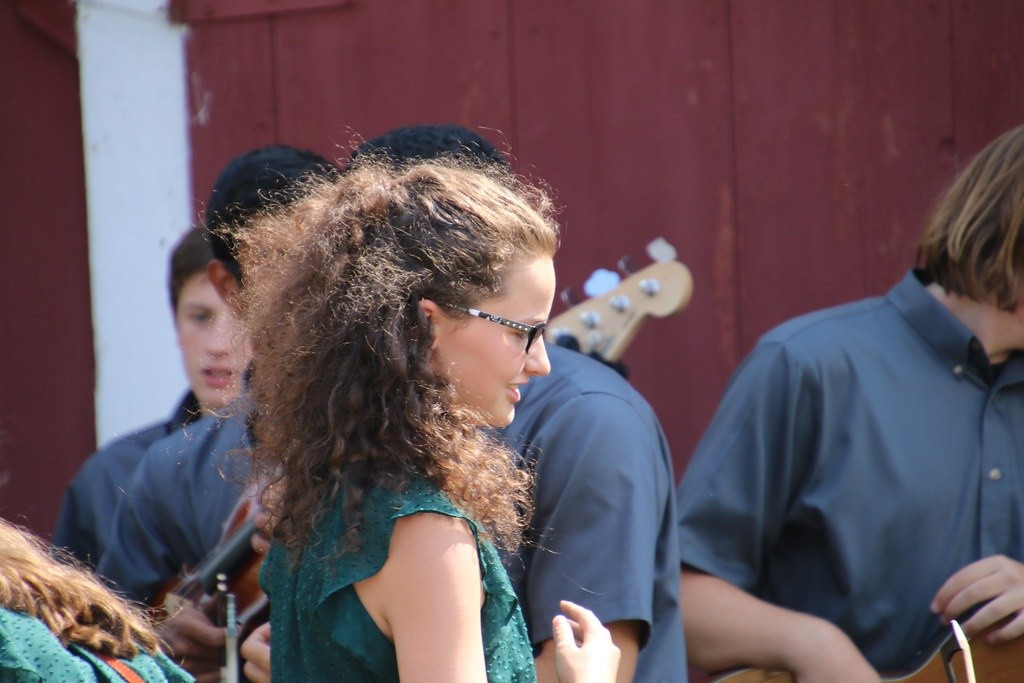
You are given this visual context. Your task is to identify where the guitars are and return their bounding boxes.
[150,235,696,683]
[689,604,1024,683]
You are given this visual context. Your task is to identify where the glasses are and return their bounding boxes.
[445,302,548,355]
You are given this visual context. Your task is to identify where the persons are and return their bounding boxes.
[675,124,1024,683]
[0,125,686,683]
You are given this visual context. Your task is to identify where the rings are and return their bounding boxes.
[180,658,185,667]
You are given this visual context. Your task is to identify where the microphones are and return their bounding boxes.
[200,511,270,598]
